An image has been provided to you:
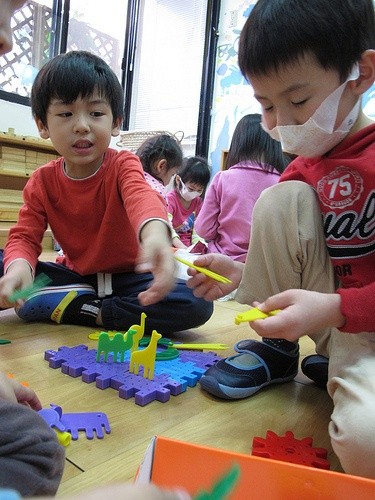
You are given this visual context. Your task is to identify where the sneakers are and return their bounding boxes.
[301,355,330,389]
[201,338,300,400]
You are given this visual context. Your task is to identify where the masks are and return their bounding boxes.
[258,63,363,160]
[178,178,202,202]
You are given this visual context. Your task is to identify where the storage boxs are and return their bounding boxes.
[132,434,375,499]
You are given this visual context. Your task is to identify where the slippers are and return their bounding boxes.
[14,284,95,325]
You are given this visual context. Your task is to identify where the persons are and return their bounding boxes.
[167,156,212,253]
[0,0,27,57]
[134,134,183,199]
[199,0,375,477]
[0,51,214,331]
[195,113,292,263]
[0,374,182,500]
